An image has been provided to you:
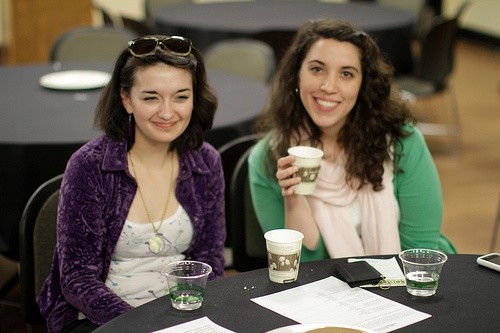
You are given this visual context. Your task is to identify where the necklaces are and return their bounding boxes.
[128,149,174,255]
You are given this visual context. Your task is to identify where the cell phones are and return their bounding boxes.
[476,253,500,272]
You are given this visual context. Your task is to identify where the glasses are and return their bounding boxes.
[126,35,193,58]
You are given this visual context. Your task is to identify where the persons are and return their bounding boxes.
[24,34,228,333]
[247,17,458,270]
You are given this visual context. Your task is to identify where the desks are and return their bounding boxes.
[0,63,270,263]
[96,254,500,333]
[156,0,419,80]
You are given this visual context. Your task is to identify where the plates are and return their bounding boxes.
[39,69,112,89]
[264,322,376,333]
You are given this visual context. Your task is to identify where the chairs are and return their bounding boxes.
[204,40,277,82]
[20,174,63,333]
[50,27,134,61]
[218,132,268,272]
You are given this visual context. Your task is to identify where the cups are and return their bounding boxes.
[399,248,447,296]
[264,229,302,284]
[286,146,324,197]
[161,261,212,311]
